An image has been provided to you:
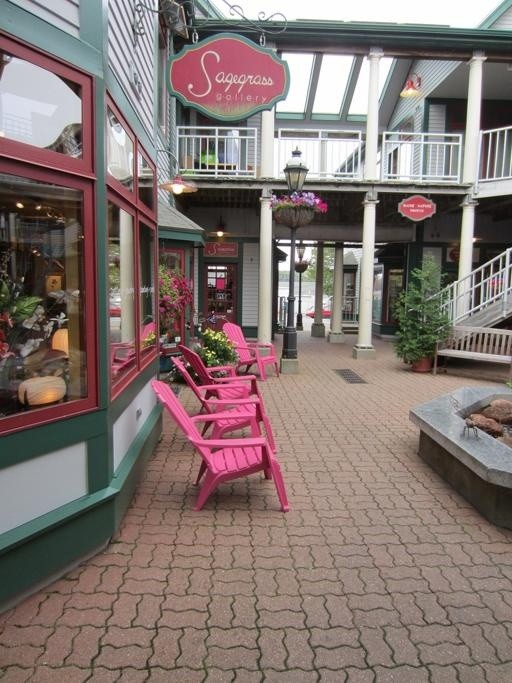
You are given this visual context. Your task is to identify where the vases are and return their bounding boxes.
[161,335,167,344]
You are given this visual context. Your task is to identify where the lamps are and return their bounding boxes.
[217,216,224,237]
[400,73,420,98]
[158,150,198,195]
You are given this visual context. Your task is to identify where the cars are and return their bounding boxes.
[109,290,123,316]
[304,303,331,318]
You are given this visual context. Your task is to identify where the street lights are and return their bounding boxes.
[279,144,311,360]
[293,237,308,331]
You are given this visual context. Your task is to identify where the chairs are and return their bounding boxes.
[151,345,291,513]
[223,323,280,381]
[110,322,156,370]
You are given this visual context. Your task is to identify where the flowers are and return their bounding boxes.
[159,263,194,334]
[139,330,155,348]
[0,237,69,376]
[270,191,328,214]
[201,327,240,364]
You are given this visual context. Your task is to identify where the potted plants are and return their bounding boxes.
[391,254,453,373]
